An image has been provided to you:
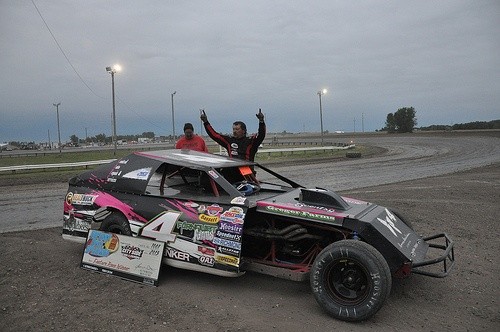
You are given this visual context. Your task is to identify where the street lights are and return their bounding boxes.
[171,91,177,140]
[52,102,62,152]
[317,89,327,142]
[106,65,120,151]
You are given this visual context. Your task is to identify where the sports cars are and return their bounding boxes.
[62,149,455,322]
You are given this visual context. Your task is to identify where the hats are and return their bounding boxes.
[184,123,193,132]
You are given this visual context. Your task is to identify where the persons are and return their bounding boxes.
[176,123,208,152]
[201,109,266,181]
